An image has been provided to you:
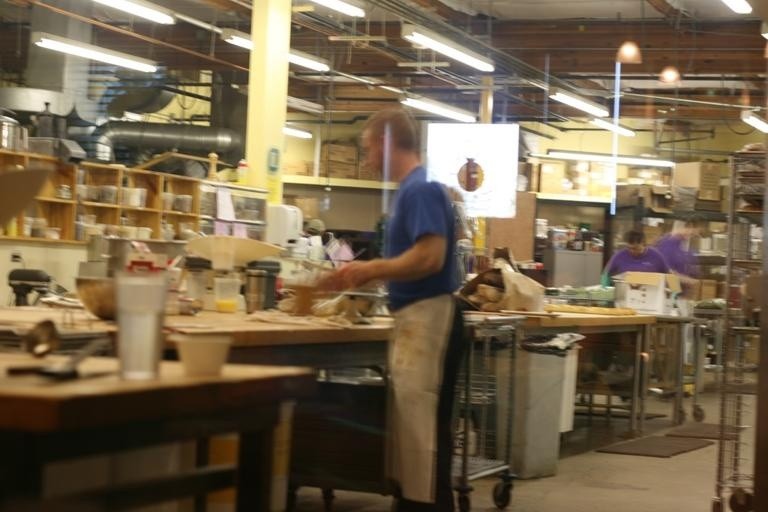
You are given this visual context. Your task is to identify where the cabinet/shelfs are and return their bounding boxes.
[711,143,767,509]
[0,148,270,246]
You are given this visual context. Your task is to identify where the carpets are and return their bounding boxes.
[595,421,745,457]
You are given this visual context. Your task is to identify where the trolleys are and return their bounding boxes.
[452,323,521,511]
[649,312,706,425]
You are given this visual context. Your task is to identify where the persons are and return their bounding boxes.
[601,229,672,275]
[287,108,466,511]
[657,214,711,276]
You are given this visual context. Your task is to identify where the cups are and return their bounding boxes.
[214,277,242,313]
[113,268,168,380]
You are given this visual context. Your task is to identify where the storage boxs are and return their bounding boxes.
[616,183,678,215]
[612,270,680,313]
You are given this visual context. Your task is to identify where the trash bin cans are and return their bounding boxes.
[495,334,571,481]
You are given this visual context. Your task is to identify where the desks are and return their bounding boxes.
[461,305,705,441]
[0,314,394,512]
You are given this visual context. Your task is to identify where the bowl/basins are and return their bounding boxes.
[168,336,233,375]
